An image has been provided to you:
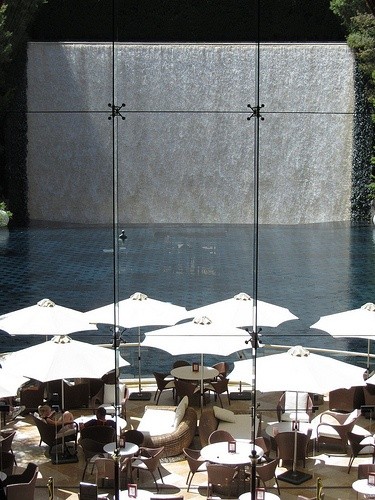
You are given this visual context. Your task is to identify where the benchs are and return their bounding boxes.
[200,410,262,449]
[138,405,196,460]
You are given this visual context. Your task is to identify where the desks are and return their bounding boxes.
[102,440,139,485]
[73,415,126,433]
[265,421,320,458]
[171,366,219,401]
[350,478,375,500]
[201,441,264,489]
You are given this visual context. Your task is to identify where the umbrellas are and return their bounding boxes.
[0,292,375,476]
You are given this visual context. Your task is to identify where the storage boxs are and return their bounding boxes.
[368,472,375,485]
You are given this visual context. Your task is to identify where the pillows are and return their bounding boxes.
[285,392,307,413]
[103,384,125,406]
[213,406,235,424]
[174,396,189,430]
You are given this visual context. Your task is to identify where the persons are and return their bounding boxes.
[80,407,115,431]
[38,405,74,434]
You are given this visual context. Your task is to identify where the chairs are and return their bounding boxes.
[0,359,375,500]
